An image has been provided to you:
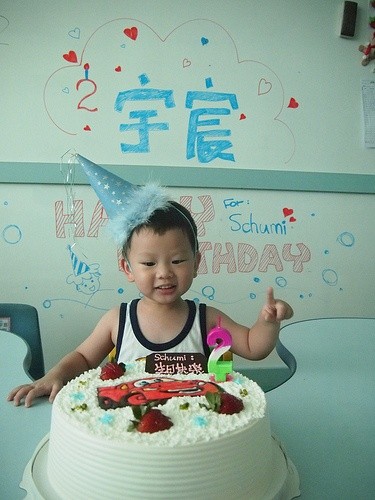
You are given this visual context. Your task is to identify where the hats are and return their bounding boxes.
[75,153,170,246]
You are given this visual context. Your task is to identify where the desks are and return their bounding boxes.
[0,317,375,500]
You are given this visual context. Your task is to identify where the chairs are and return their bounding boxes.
[0,302,45,379]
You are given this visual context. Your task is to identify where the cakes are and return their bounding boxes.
[45,351,271,500]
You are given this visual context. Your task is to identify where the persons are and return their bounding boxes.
[7,148,294,408]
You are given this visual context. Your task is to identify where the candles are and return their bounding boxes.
[206,313,234,382]
